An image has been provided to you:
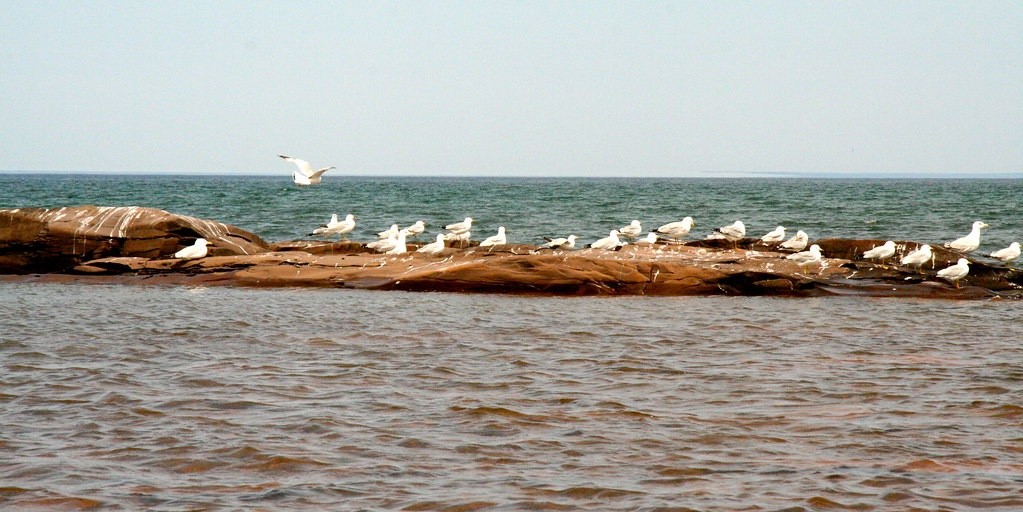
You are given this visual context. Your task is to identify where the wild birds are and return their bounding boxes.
[359,217,507,254]
[709,220,746,250]
[750,225,825,275]
[275,153,337,187]
[163,237,213,260]
[850,220,1022,289]
[532,234,579,253]
[305,213,356,244]
[581,215,695,252]
